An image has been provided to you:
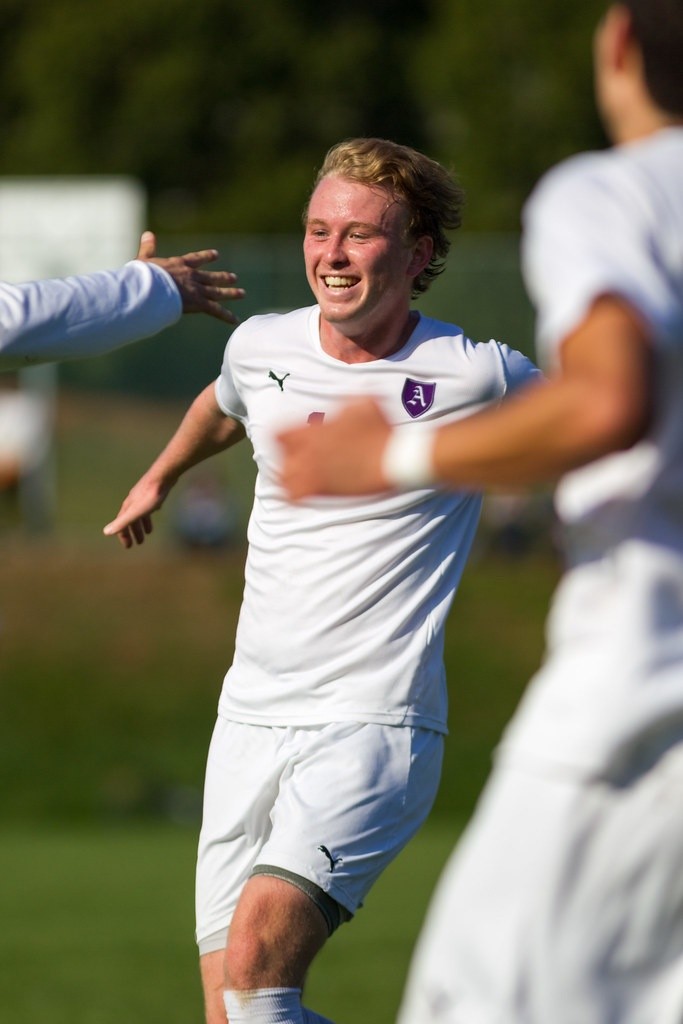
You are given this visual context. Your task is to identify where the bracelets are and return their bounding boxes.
[384,427,432,488]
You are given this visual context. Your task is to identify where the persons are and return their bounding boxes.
[102,137,543,1024]
[0,372,54,511]
[275,0,683,1024]
[1,231,247,374]
